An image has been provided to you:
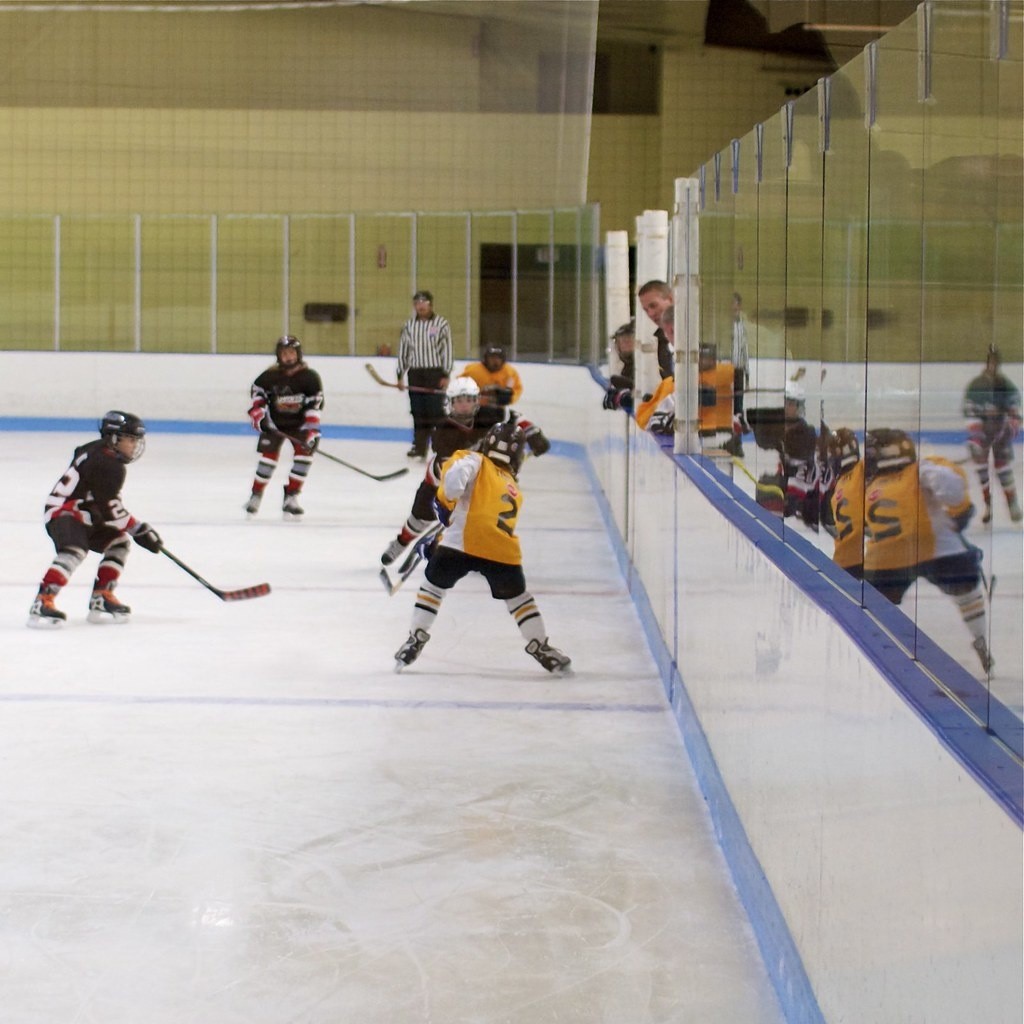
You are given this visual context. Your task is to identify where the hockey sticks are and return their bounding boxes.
[366,363,447,395]
[378,521,443,596]
[983,567,997,602]
[161,545,271,602]
[275,429,409,481]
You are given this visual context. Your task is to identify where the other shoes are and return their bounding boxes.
[406,443,428,464]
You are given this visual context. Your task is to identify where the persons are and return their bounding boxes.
[602,280,675,435]
[962,344,1023,530]
[732,293,750,416]
[732,383,996,680]
[381,377,550,567]
[463,342,523,409]
[699,343,750,458]
[394,423,574,677]
[27,411,164,629]
[396,290,453,463]
[247,336,325,520]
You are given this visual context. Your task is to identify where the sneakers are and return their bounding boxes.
[283,483,305,522]
[983,500,994,524]
[525,637,571,676]
[1009,501,1023,524]
[396,627,431,671]
[29,585,67,629]
[972,637,998,679]
[86,578,131,624]
[247,492,262,517]
[381,536,407,566]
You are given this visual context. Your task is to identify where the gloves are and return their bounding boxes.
[603,385,633,409]
[249,405,275,433]
[133,524,163,554]
[651,412,675,434]
[302,429,319,455]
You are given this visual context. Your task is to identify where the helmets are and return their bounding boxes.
[783,381,809,422]
[615,325,634,364]
[481,343,507,370]
[100,409,145,463]
[275,335,302,365]
[868,426,915,469]
[827,428,860,471]
[443,377,481,421]
[484,424,525,468]
